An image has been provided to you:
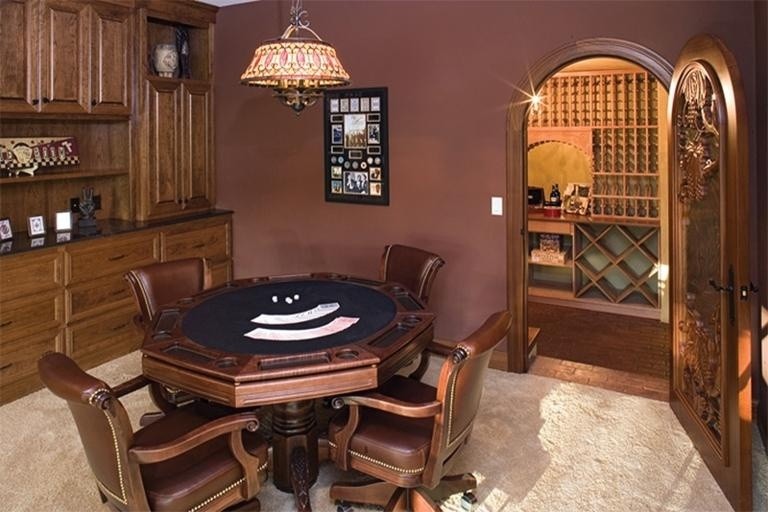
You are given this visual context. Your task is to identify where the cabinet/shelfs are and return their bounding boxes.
[528,208,582,300]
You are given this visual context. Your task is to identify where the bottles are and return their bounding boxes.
[550,182,560,206]
[155,43,177,79]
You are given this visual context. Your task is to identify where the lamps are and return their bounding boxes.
[238,0,352,117]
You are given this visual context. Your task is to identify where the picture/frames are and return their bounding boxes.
[324,87,389,206]
